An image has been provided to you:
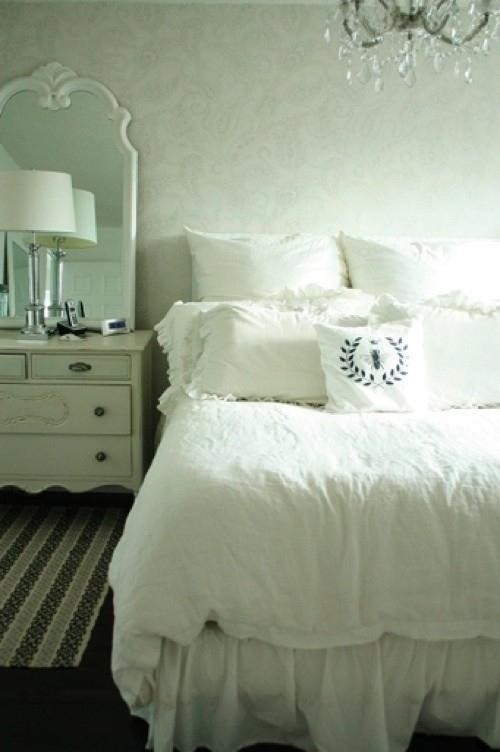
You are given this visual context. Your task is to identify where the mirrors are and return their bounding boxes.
[0,62,140,331]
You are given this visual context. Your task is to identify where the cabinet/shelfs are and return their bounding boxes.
[0,329,155,499]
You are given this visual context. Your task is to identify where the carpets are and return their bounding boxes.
[0,503,129,668]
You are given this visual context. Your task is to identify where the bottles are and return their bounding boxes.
[0,284,9,317]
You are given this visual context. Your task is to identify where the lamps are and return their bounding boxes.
[0,169,77,340]
[323,0,500,93]
[22,189,97,317]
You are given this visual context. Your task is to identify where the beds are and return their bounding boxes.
[105,261,499,748]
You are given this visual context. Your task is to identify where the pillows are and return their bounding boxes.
[155,283,374,405]
[312,313,428,416]
[184,225,350,301]
[340,228,499,309]
[420,291,499,413]
[181,304,370,400]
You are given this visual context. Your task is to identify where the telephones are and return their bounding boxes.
[57,299,87,336]
